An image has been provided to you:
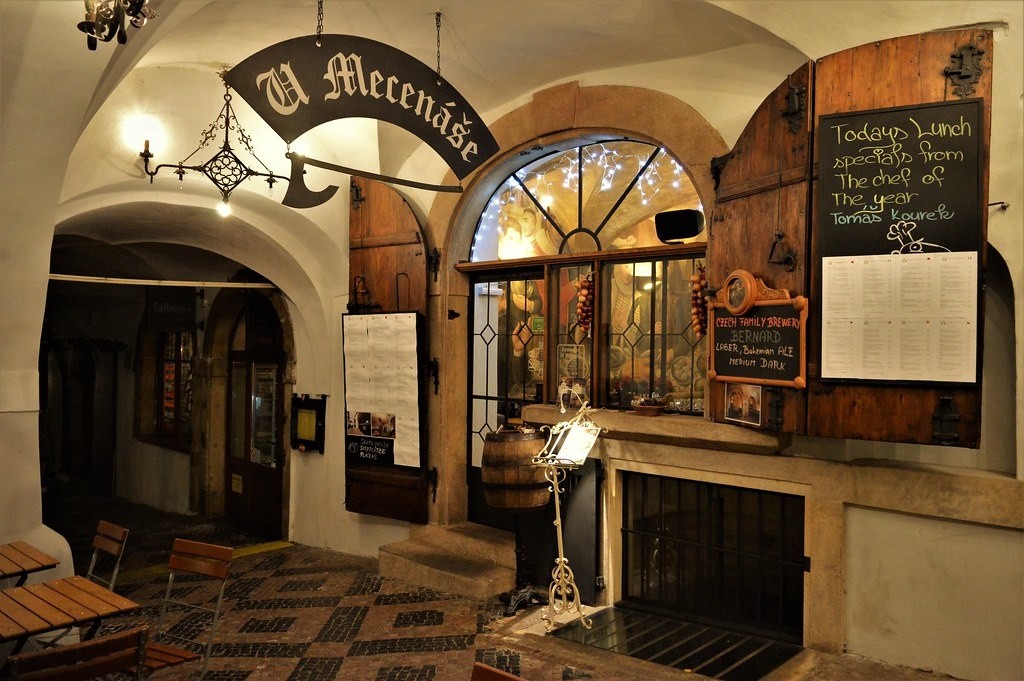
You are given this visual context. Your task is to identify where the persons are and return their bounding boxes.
[722,380,762,428]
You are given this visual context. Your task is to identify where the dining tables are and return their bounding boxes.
[0,537,60,588]
[0,572,139,680]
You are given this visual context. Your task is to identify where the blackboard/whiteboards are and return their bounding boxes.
[345,436,394,475]
[707,299,806,386]
[815,98,984,390]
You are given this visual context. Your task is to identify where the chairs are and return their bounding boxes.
[105,537,237,680]
[45,518,133,654]
[3,627,154,681]
